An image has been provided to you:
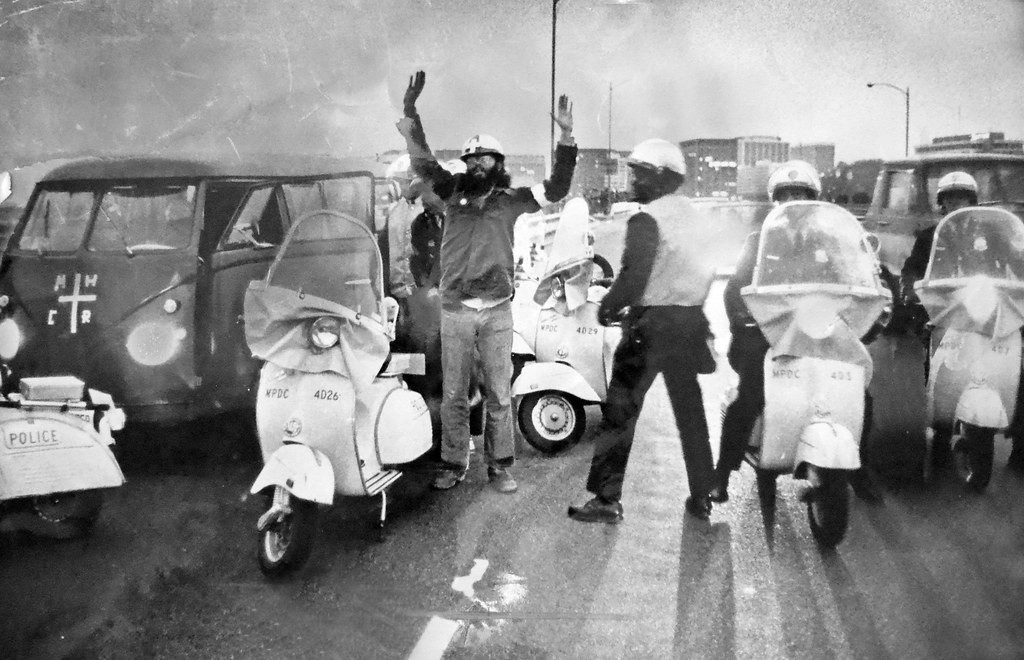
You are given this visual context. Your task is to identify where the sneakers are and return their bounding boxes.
[433,466,468,489]
[487,465,518,492]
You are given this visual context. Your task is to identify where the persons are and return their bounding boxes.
[566,139,720,524]
[363,71,578,493]
[898,169,1024,471]
[708,158,885,504]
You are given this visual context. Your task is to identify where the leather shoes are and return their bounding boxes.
[568,496,624,523]
[687,496,712,517]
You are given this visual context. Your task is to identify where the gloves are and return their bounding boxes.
[902,288,921,307]
[729,312,748,333]
[598,303,625,326]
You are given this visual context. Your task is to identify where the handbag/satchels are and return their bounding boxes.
[693,335,717,374]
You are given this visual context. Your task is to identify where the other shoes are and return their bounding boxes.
[710,485,729,501]
[848,470,877,503]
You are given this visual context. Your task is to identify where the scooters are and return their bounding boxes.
[739,195,895,547]
[910,204,1023,496]
[509,196,635,460]
[235,208,491,582]
[0,371,132,554]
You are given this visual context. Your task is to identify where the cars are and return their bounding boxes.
[1,147,769,441]
[856,146,1023,340]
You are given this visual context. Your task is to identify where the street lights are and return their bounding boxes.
[867,81,910,157]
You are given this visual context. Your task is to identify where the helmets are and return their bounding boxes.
[766,159,822,200]
[628,139,688,174]
[459,135,505,163]
[934,172,979,212]
